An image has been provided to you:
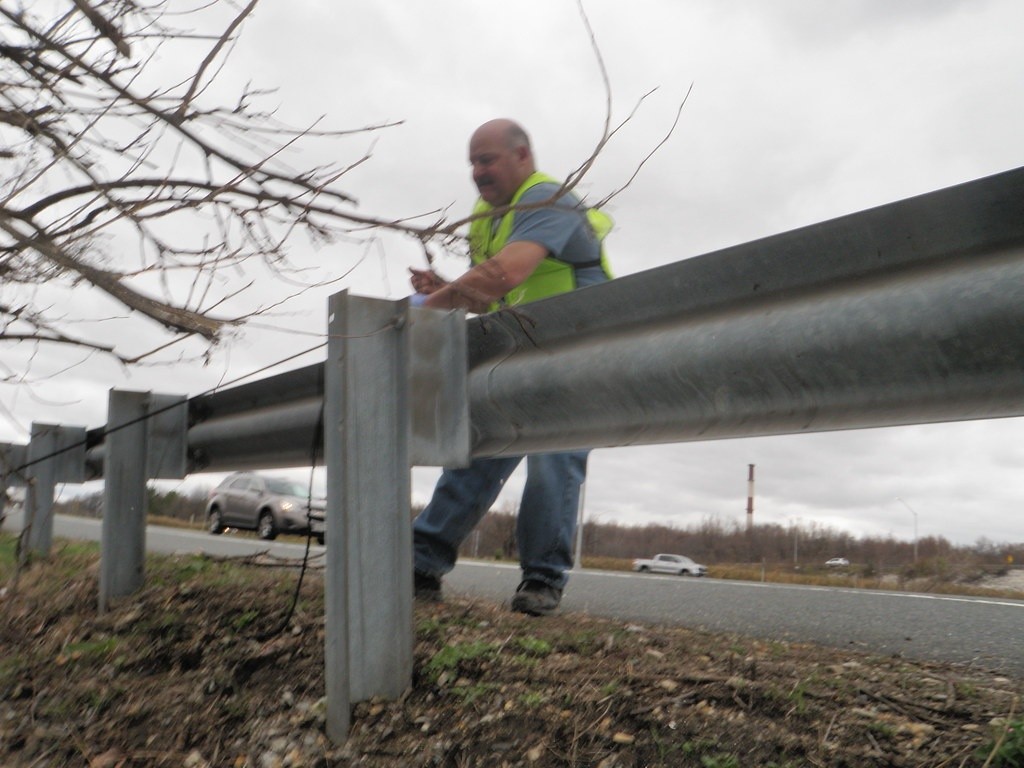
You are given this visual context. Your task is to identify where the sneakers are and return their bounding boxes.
[413,572,443,600]
[511,580,563,615]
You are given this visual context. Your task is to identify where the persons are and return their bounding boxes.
[406,118,612,617]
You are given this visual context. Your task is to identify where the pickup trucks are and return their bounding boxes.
[631,553,710,578]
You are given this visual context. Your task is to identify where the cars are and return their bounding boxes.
[205,469,326,545]
